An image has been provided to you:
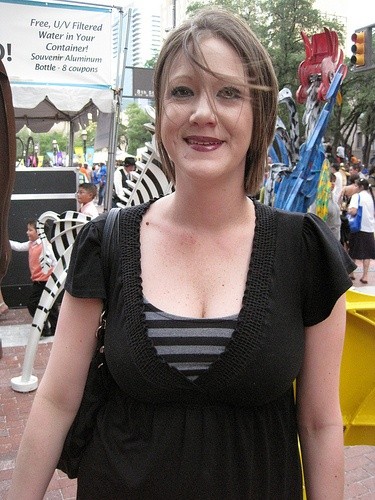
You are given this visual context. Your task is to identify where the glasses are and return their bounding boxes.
[77,192,89,194]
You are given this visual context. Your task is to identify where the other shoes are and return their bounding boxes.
[360,279,367,284]
[350,277,355,280]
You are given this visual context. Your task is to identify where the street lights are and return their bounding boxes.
[23,150,26,165]
[80,129,88,161]
[52,140,57,166]
[33,145,38,166]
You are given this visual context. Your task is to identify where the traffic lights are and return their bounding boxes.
[351,31,364,66]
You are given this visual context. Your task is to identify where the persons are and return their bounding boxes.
[72,157,136,220]
[7,8,358,500]
[9,218,59,337]
[257,135,375,254]
[345,178,375,284]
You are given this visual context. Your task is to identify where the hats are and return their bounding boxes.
[123,157,136,166]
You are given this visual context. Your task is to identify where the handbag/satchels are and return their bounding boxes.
[58,209,120,478]
[347,193,362,232]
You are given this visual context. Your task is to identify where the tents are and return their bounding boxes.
[92,147,137,164]
